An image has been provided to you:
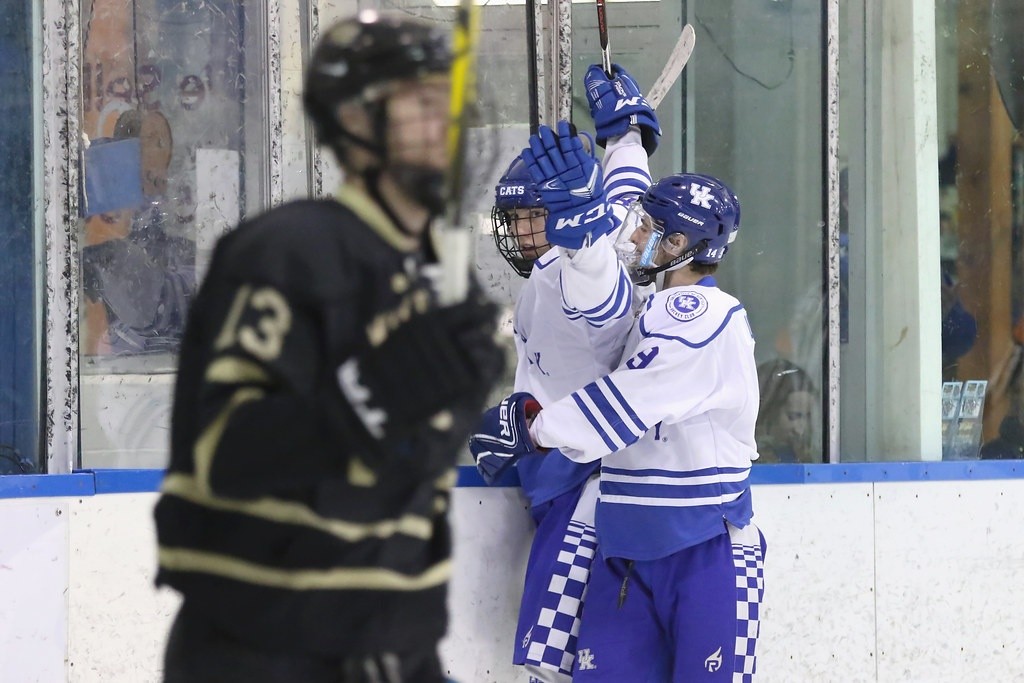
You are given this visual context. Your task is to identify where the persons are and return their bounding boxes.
[153,10,513,683]
[467,174,767,683]
[490,64,663,683]
[754,360,820,464]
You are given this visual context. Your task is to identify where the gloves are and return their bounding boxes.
[333,278,508,443]
[521,120,622,250]
[584,62,662,157]
[468,392,543,486]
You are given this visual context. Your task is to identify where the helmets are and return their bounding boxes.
[303,12,456,143]
[491,154,556,279]
[637,172,740,261]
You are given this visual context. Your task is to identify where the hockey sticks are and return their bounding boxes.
[645,23,696,111]
[446,0,484,310]
[595,0,615,80]
